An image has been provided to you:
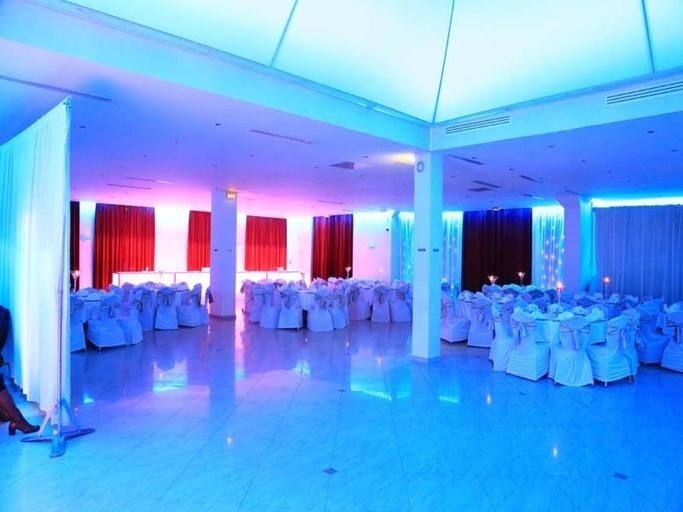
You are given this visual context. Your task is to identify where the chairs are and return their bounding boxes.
[547,304,563,313]
[70,295,86,354]
[584,305,608,319]
[87,294,126,352]
[488,304,515,372]
[502,284,520,292]
[482,284,494,297]
[370,285,390,322]
[117,289,144,345]
[249,284,264,324]
[636,306,669,367]
[277,288,304,331]
[259,285,281,329]
[621,308,641,381]
[391,282,411,321]
[531,298,549,312]
[202,286,212,326]
[528,289,544,301]
[662,310,683,372]
[347,282,371,320]
[546,289,559,303]
[586,315,634,387]
[306,289,333,332]
[504,311,549,382]
[441,291,470,344]
[467,297,494,348]
[178,284,201,329]
[136,289,154,332]
[547,316,594,387]
[502,289,517,298]
[329,286,348,330]
[643,301,659,313]
[153,287,178,327]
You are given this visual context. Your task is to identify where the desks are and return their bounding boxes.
[78,296,103,323]
[534,318,609,346]
[300,288,315,312]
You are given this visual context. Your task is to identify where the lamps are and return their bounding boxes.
[517,272,526,285]
[70,270,80,292]
[488,275,499,284]
[344,264,352,279]
[556,280,565,303]
[603,276,611,299]
